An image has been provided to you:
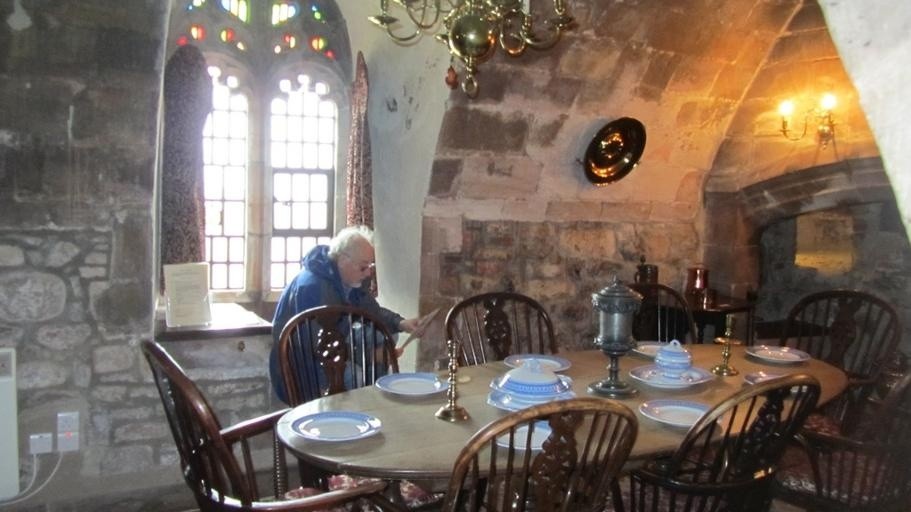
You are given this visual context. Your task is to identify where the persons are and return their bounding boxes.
[269,224,429,487]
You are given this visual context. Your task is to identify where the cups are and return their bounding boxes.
[633,264,658,300]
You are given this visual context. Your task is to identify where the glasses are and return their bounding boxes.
[346,252,375,271]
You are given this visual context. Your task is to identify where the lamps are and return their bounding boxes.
[366,0,578,101]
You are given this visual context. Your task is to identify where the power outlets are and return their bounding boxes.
[55,411,80,452]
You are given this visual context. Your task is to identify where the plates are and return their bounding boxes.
[628,340,669,358]
[483,374,577,414]
[374,373,449,398]
[636,399,723,428]
[502,352,573,374]
[488,421,553,451]
[627,363,715,391]
[289,410,382,442]
[741,371,814,393]
[743,344,812,367]
